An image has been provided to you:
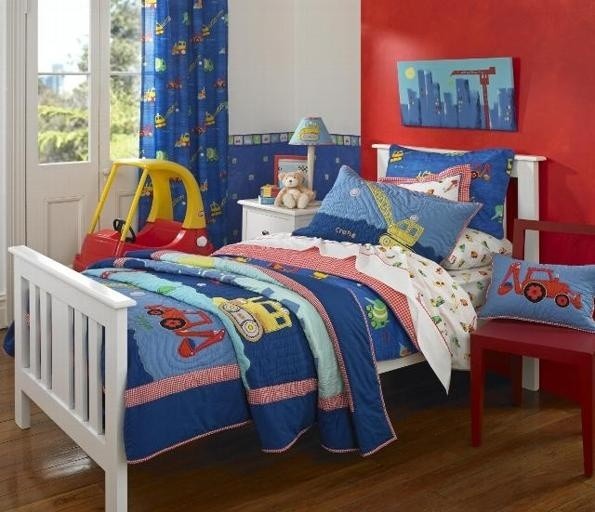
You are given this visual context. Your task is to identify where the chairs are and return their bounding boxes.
[467,209,594,478]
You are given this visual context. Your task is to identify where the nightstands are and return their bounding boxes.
[236,192,319,244]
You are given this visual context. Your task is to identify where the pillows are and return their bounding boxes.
[377,165,471,207]
[291,163,483,267]
[481,249,594,329]
[385,144,514,240]
[440,226,512,272]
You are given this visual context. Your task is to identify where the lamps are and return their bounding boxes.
[286,116,334,194]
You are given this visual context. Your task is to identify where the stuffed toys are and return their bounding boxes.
[274,171,315,209]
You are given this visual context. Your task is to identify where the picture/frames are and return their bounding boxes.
[273,154,311,190]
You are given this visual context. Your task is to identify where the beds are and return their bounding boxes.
[7,140,546,511]
